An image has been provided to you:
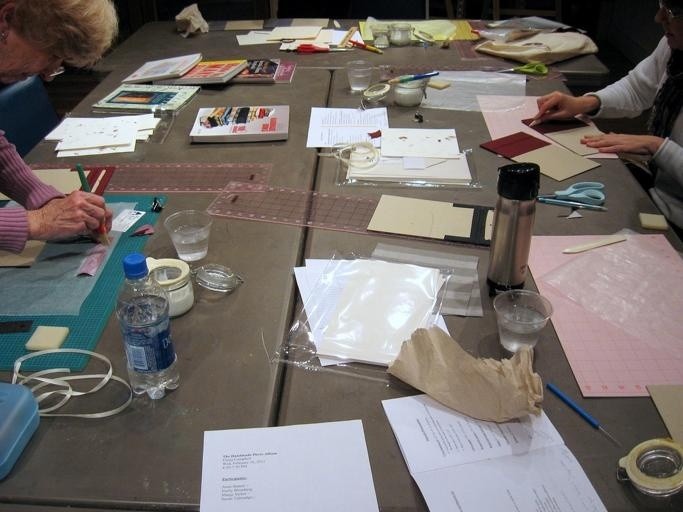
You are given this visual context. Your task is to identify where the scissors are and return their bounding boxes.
[296,44,352,54]
[498,61,548,75]
[536,182,605,206]
[266,39,295,43]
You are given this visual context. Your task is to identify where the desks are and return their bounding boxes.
[88,10,612,96]
[272,66,682,510]
[0,65,332,510]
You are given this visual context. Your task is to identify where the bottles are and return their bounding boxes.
[486,161,540,299]
[144,258,194,320]
[395,75,424,107]
[618,437,682,500]
[116,252,180,399]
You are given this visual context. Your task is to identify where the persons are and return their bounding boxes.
[1,0,121,256]
[534,2,681,237]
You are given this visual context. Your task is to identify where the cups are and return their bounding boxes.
[164,209,212,261]
[493,291,552,352]
[347,60,373,93]
[369,22,413,49]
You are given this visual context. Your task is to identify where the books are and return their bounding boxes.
[273,59,297,83]
[346,146,473,186]
[123,52,203,84]
[188,106,289,143]
[232,56,281,84]
[151,58,248,86]
[224,17,332,51]
[94,76,201,115]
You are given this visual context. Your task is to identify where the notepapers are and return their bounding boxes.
[25,325,70,350]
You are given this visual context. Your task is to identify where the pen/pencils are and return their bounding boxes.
[529,119,541,127]
[348,40,383,55]
[414,31,436,43]
[90,169,106,193]
[76,162,111,245]
[547,382,623,447]
[387,71,439,83]
[538,198,608,212]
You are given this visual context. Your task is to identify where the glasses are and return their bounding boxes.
[46,50,65,77]
[658,1,683,24]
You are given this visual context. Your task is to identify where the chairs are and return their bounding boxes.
[0,72,62,161]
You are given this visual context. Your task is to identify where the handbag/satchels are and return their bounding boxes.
[474,28,599,66]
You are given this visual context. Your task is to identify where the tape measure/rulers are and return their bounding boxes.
[338,26,358,48]
[0,320,33,333]
